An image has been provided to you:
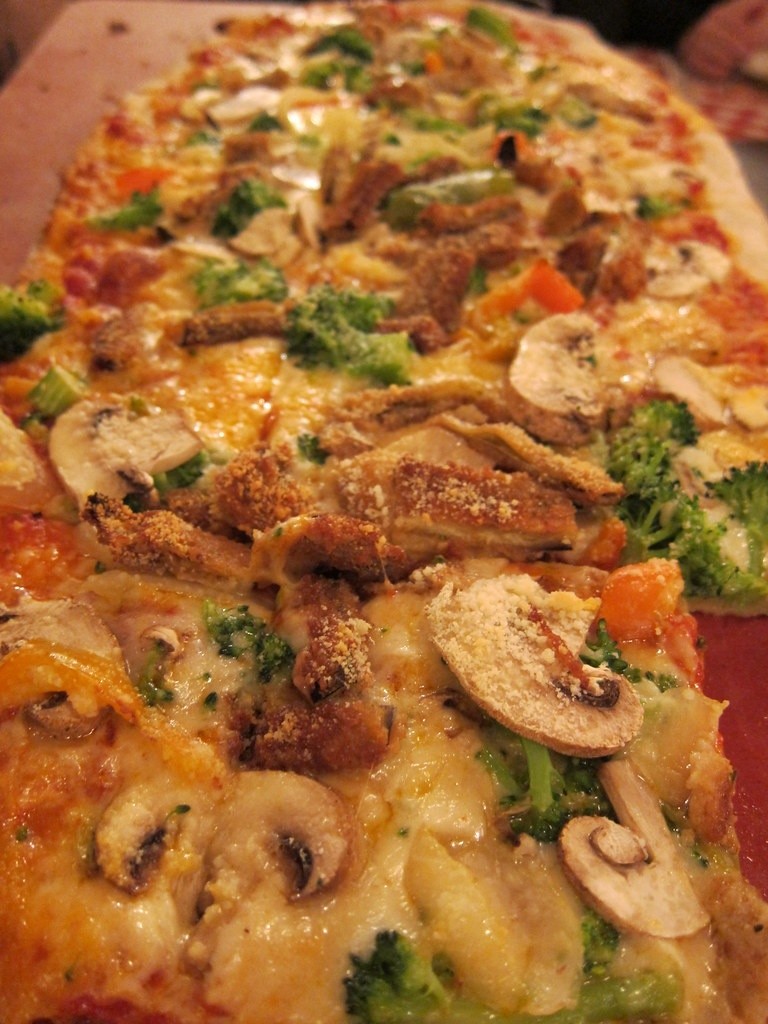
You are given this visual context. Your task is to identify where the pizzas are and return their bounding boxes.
[0,508,768,1024]
[0,0,767,616]
